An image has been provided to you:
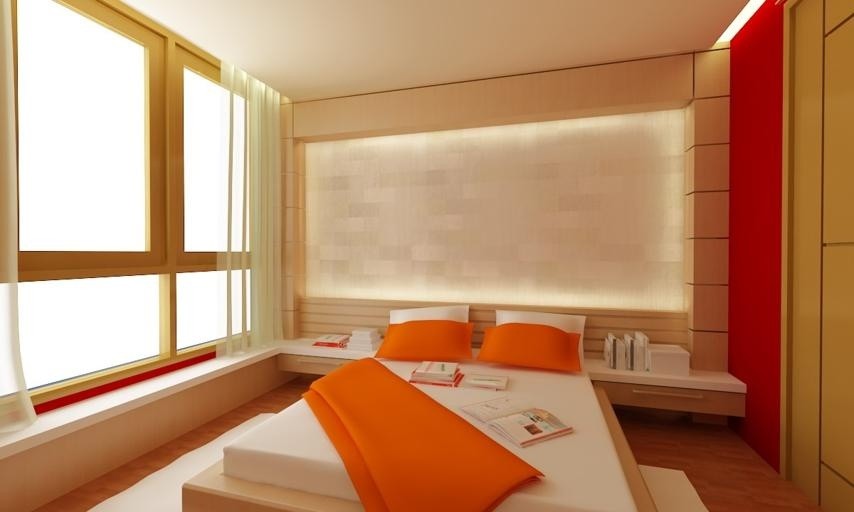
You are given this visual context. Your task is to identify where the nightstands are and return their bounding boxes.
[276,338,384,375]
[585,356,748,419]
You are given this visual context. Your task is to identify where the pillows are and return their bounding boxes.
[493,309,586,368]
[388,305,470,326]
[374,317,474,363]
[477,323,581,374]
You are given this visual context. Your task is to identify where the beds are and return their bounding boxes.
[181,295,692,512]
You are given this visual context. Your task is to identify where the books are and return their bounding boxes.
[310,326,382,354]
[408,360,509,393]
[601,328,650,374]
[461,395,575,450]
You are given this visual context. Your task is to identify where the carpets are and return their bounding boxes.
[80,412,706,512]
